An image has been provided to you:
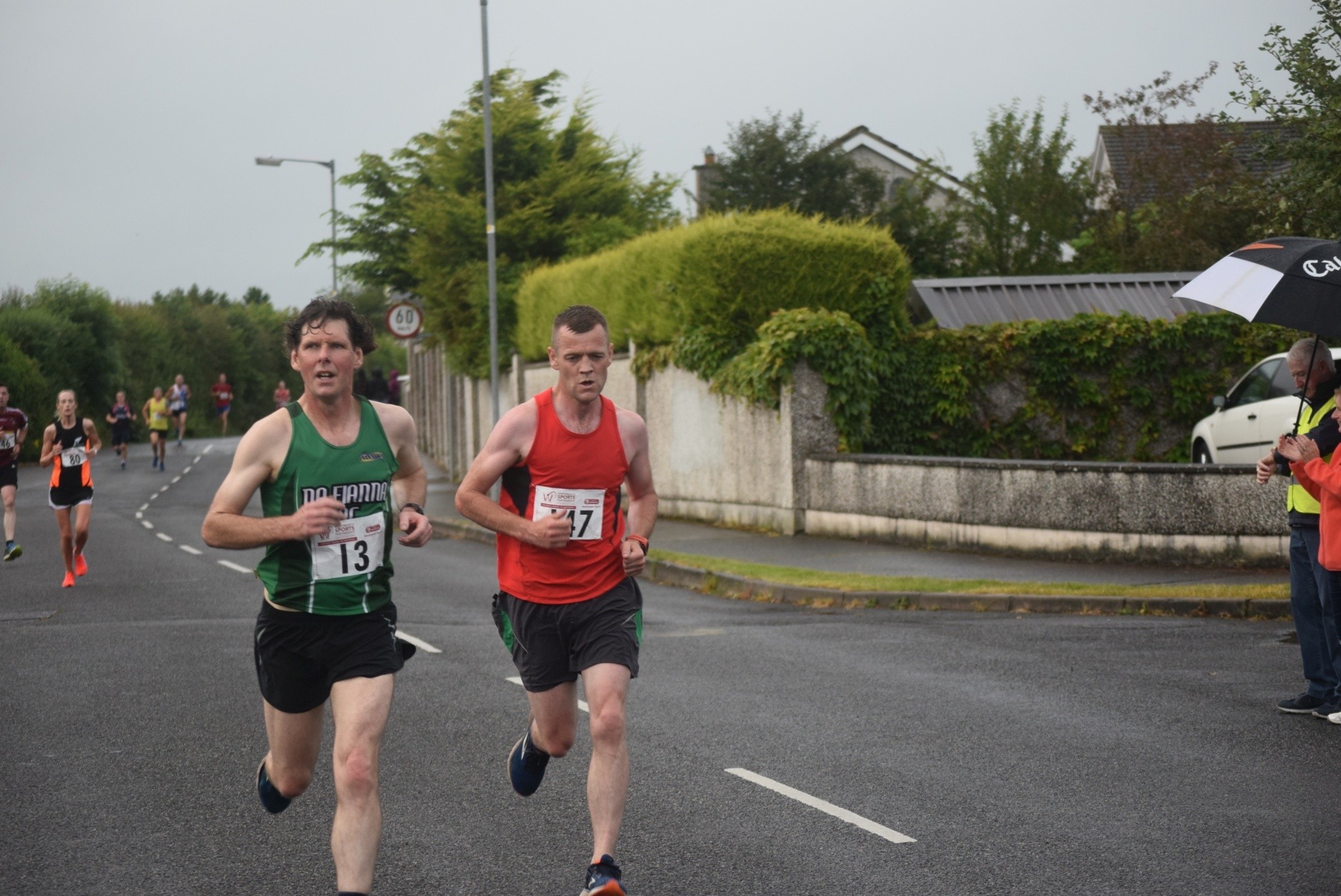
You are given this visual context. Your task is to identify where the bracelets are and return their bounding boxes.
[400,503,424,515]
[628,534,649,556]
[93,448,97,454]
[16,442,23,446]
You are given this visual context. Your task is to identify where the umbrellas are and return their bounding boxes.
[1169,237,1341,439]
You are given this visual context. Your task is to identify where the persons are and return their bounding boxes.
[210,372,233,437]
[454,304,659,896]
[165,373,193,447]
[1255,339,1340,718]
[1277,374,1341,723]
[0,384,29,562]
[106,390,137,470]
[273,379,291,410]
[40,388,102,588]
[142,384,173,472]
[201,297,435,895]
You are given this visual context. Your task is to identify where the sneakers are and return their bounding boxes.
[508,709,553,798]
[73,546,88,577]
[579,855,628,896]
[3,543,23,561]
[62,572,75,587]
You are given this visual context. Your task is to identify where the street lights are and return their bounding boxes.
[254,156,338,294]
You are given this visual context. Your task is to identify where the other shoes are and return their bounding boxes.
[153,457,158,467]
[116,449,121,456]
[160,463,165,471]
[1327,712,1341,724]
[1311,697,1341,719]
[1276,691,1326,714]
[256,755,295,814]
[121,463,126,470]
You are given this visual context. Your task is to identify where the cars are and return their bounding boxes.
[1191,348,1341,465]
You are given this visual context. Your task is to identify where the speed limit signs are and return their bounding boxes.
[385,302,423,339]
